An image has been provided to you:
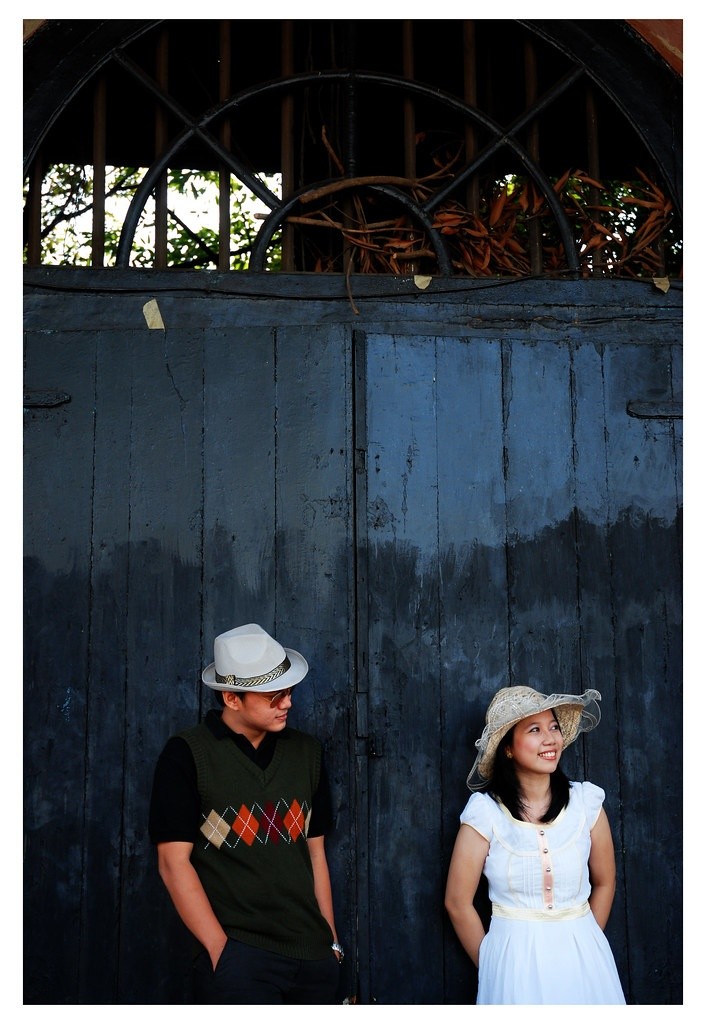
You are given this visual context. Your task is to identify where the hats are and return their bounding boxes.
[201,623,309,693]
[466,685,602,794]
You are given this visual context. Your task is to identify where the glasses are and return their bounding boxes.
[257,685,296,708]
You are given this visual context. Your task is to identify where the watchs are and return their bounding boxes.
[332,943,344,964]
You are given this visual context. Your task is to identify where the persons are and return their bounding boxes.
[150,623,352,1005]
[445,686,626,1005]
